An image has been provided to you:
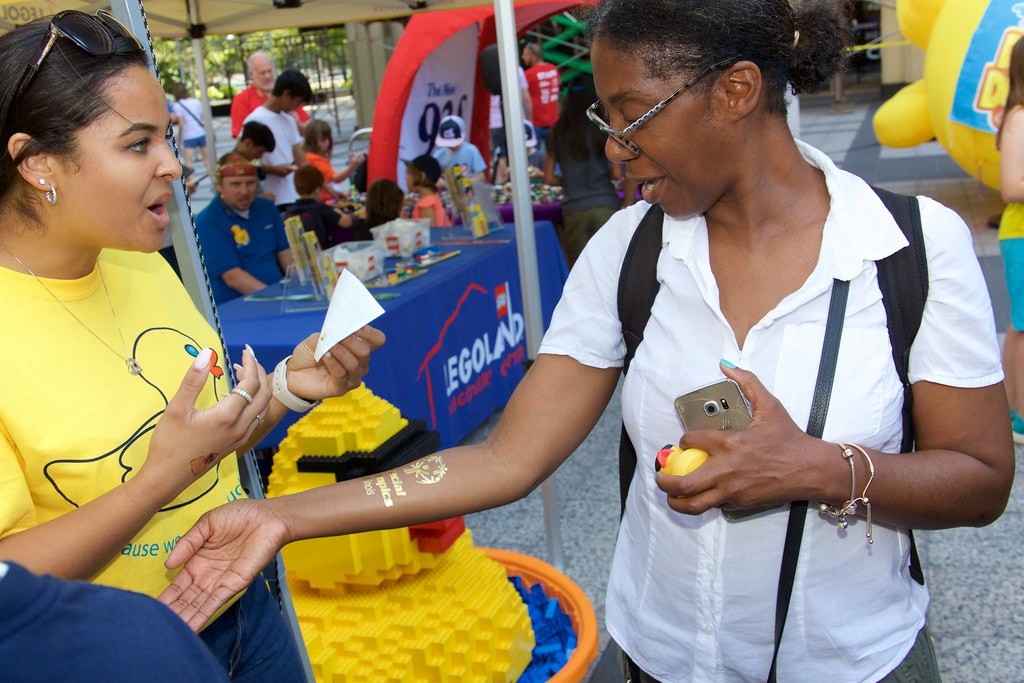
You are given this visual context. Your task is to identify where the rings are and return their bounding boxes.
[232,388,254,406]
[256,414,265,427]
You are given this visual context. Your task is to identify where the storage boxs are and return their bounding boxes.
[370,214,432,260]
[323,237,386,284]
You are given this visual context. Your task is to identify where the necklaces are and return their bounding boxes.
[2,235,146,376]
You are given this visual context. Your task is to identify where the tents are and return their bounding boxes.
[0,0,566,573]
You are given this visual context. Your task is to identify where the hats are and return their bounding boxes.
[523,119,537,148]
[400,155,441,181]
[434,115,466,148]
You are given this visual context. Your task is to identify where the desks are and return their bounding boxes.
[217,219,571,447]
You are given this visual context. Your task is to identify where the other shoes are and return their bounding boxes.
[1009,410,1024,444]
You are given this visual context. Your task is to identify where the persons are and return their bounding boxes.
[0,11,387,683]
[161,37,560,309]
[152,1,1019,683]
[992,37,1023,445]
[542,70,636,266]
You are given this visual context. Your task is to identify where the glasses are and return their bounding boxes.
[585,56,742,158]
[0,9,145,138]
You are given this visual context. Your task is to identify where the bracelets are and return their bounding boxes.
[817,440,879,549]
[270,354,319,414]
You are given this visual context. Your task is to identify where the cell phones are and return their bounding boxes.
[676,380,785,521]
